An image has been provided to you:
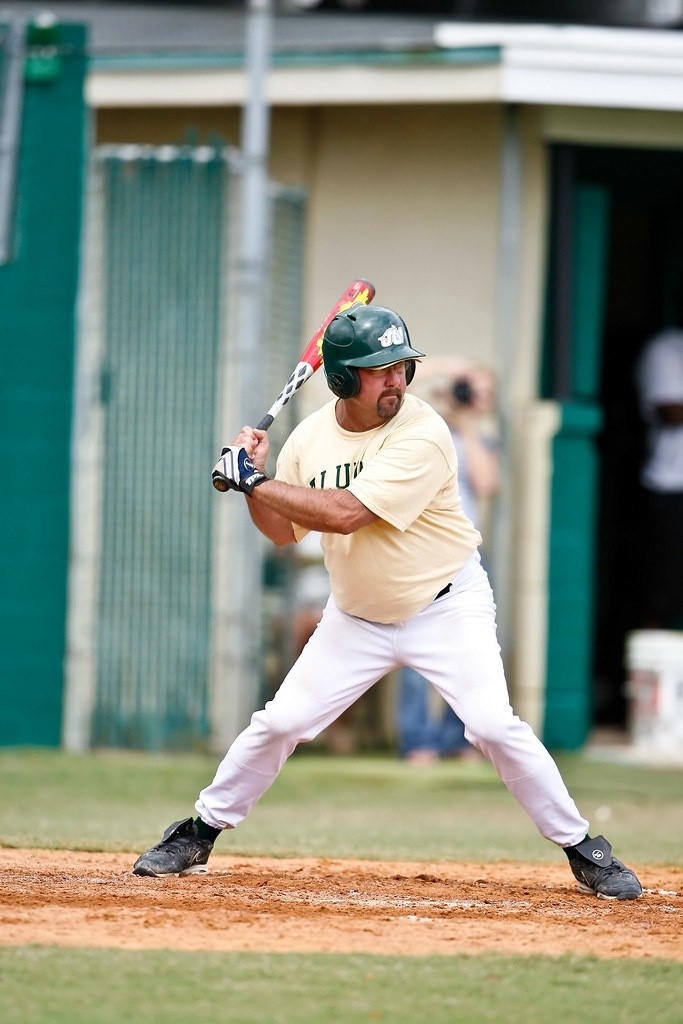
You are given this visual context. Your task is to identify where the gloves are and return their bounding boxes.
[211,446,271,498]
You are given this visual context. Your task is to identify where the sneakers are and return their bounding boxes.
[569,835,642,900]
[132,817,214,878]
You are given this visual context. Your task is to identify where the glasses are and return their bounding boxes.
[369,361,409,378]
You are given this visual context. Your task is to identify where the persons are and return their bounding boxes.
[391,362,500,764]
[136,308,642,901]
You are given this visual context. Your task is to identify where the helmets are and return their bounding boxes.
[321,301,426,400]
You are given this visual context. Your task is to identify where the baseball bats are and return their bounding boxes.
[211,275,378,495]
